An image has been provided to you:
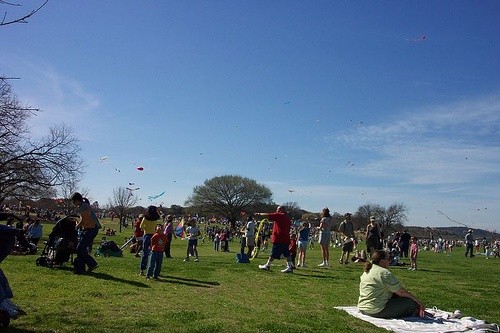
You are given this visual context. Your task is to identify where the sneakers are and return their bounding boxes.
[259,264,270,270]
[281,268,293,272]
[318,263,325,266]
[194,259,199,261]
[297,264,300,267]
[326,264,329,266]
[409,267,412,269]
[302,265,306,266]
[184,257,189,260]
[413,268,417,270]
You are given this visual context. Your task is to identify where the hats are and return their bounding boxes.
[190,219,196,222]
[370,216,376,220]
[345,212,351,216]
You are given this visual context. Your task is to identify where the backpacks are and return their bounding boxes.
[483,240,486,245]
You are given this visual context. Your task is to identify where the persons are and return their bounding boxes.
[464,228,475,258]
[26,218,42,252]
[139,206,163,276]
[383,229,411,266]
[487,240,499,261]
[23,217,34,244]
[163,214,177,258]
[245,216,256,258]
[365,215,382,258]
[146,224,170,281]
[337,212,356,264]
[482,237,488,253]
[407,236,418,271]
[288,233,296,269]
[475,238,480,254]
[356,250,425,319]
[71,192,101,275]
[419,234,461,253]
[253,205,293,273]
[316,208,332,267]
[296,223,311,267]
[133,213,144,257]
[183,219,202,262]
[240,225,246,254]
[204,225,233,252]
[7,218,17,253]
